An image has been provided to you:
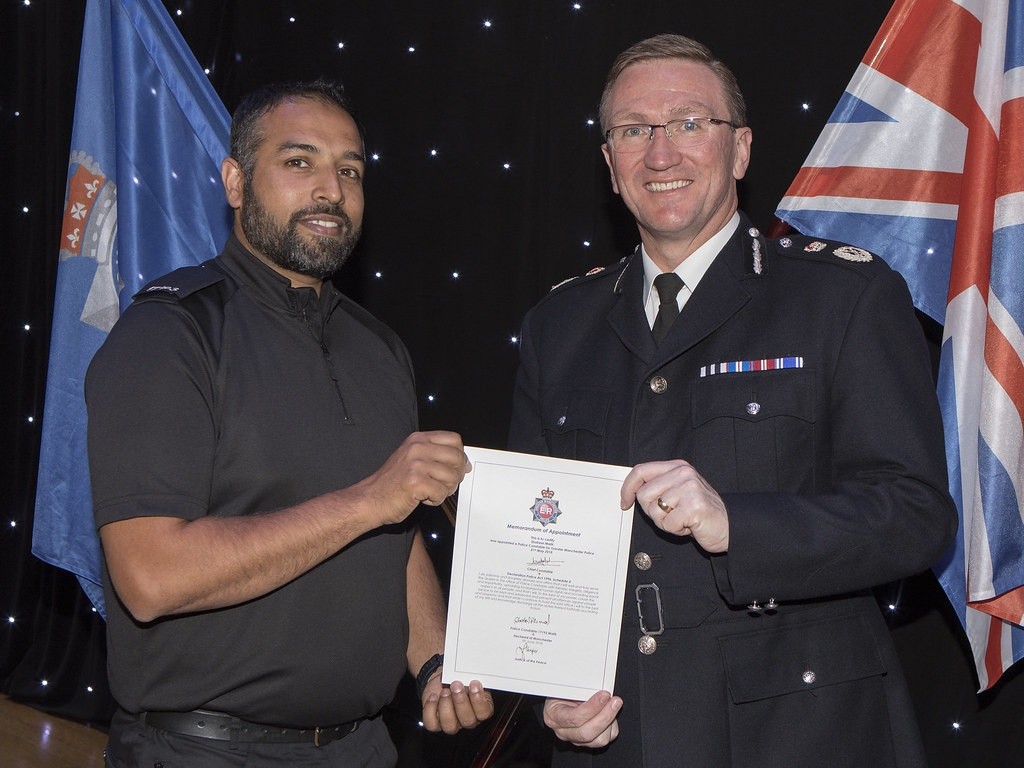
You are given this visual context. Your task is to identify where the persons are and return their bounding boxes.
[85,83,491,768]
[504,34,959,768]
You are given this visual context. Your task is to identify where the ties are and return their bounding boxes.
[651,272,685,350]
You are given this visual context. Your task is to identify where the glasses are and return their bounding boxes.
[605,115,739,154]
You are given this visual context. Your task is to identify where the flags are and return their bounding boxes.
[34,0,231,624]
[773,1,1024,694]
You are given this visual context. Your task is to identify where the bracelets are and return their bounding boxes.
[415,653,444,696]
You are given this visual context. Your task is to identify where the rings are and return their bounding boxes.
[656,497,673,513]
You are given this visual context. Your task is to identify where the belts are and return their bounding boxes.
[131,707,368,748]
